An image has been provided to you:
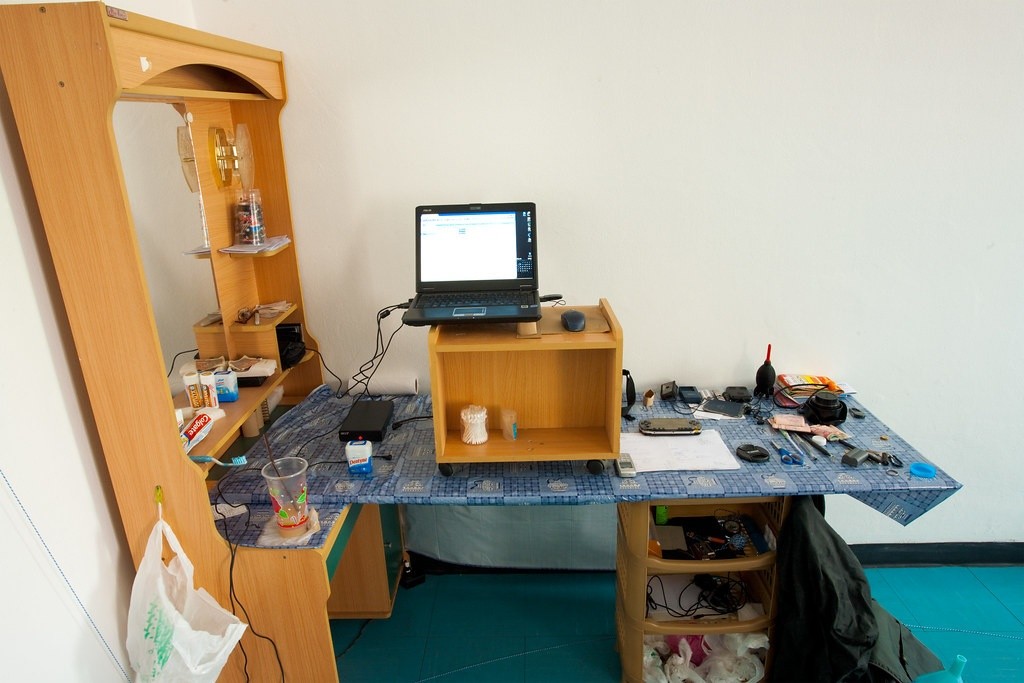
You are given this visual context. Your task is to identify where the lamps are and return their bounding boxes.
[207,122,256,192]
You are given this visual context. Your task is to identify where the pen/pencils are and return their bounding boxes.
[776,427,805,457]
[795,430,832,458]
[788,430,818,461]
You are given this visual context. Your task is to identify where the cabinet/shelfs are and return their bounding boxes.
[428,299,621,475]
[6,2,409,682]
[613,495,785,683]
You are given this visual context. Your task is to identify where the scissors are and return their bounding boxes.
[766,438,806,466]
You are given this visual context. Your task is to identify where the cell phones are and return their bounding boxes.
[616,453,637,478]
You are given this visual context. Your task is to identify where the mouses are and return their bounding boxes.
[561,309,586,332]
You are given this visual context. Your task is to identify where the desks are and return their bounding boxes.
[214,379,963,528]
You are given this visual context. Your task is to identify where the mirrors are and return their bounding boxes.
[114,101,230,410]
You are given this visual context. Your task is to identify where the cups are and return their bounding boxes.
[261,457,309,538]
[459,404,489,444]
[502,409,519,440]
[232,187,268,248]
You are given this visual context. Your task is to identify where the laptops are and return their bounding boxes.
[402,203,543,326]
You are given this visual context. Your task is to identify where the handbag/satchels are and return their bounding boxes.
[125,518,249,683]
